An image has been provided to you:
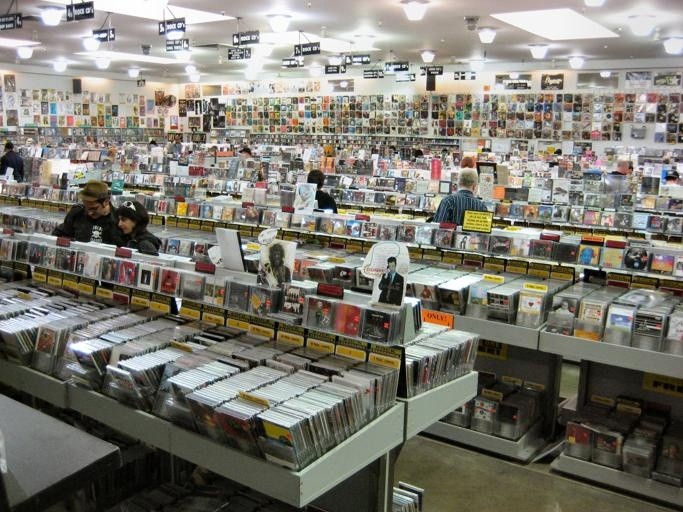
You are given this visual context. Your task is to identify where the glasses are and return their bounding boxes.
[121,201,137,211]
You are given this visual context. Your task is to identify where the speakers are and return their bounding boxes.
[73,79,81,94]
[426,74,435,91]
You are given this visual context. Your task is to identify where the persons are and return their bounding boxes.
[377,256,404,306]
[237,148,255,169]
[432,168,488,226]
[52,179,123,246]
[0,142,24,183]
[660,169,683,198]
[115,199,162,256]
[610,158,630,175]
[307,169,337,215]
[256,244,290,287]
[460,156,474,168]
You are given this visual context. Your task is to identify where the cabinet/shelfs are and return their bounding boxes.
[0,125,682,512]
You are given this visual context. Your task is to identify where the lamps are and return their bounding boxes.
[468,0,682,80]
[0,0,238,88]
[244,0,437,88]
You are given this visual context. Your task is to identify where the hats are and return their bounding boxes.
[76,179,112,202]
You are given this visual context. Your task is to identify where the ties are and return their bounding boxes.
[386,272,391,288]
